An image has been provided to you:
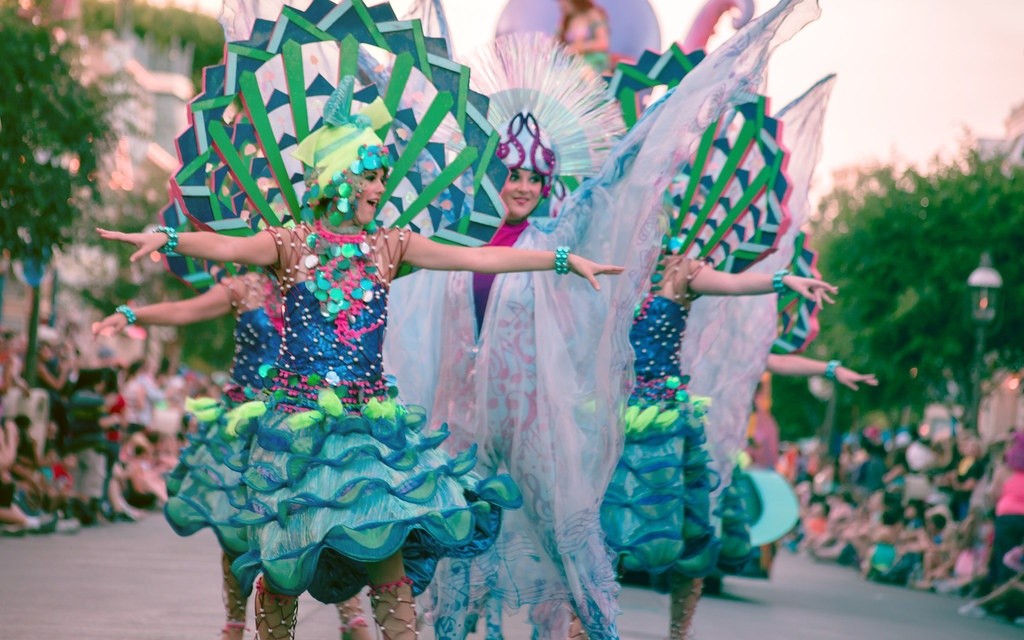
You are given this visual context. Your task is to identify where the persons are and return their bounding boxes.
[0,325,220,535]
[776,424,1024,628]
[90,0,879,640]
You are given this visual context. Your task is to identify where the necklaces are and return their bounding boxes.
[322,215,360,234]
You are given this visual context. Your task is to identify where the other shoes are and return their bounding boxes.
[0,520,23,536]
[56,515,79,532]
[24,512,56,534]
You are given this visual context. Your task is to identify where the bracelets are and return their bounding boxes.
[824,359,843,378]
[554,246,571,275]
[116,304,138,324]
[153,225,178,255]
[772,269,790,294]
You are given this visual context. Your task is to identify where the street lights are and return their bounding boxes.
[965,251,1004,433]
